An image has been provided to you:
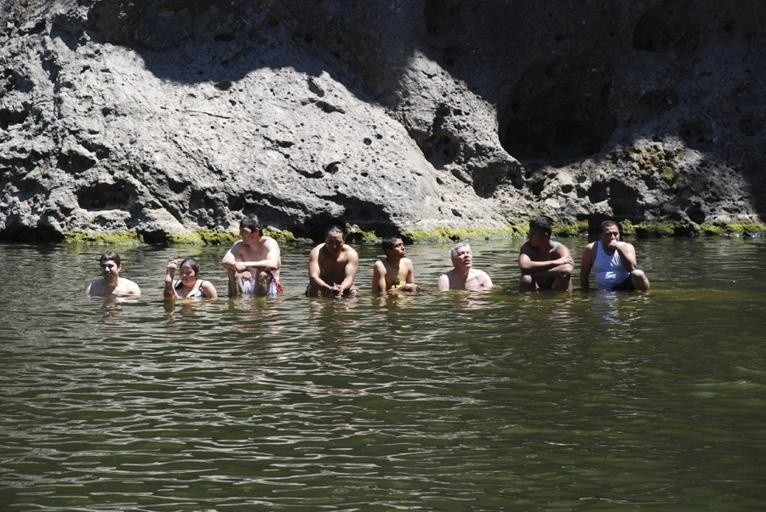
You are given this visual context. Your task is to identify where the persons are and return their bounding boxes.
[305,224,360,297]
[371,237,421,293]
[579,219,651,291]
[162,257,218,299]
[221,213,282,296]
[85,251,141,296]
[518,217,575,293]
[436,241,493,290]
